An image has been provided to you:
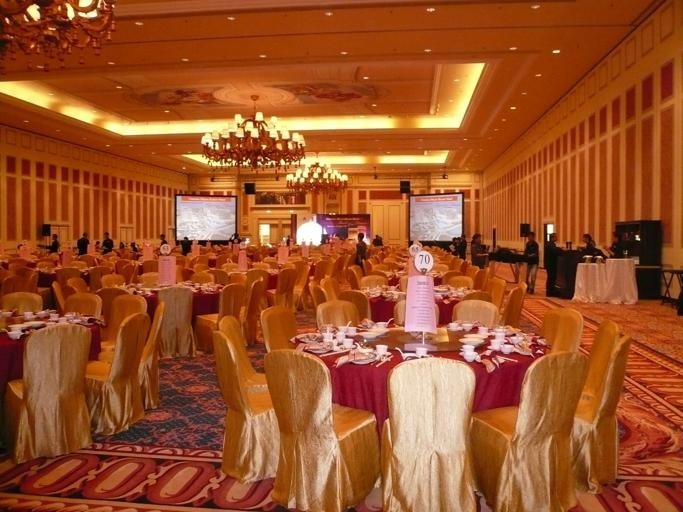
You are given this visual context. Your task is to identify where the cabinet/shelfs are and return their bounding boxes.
[611,218,658,299]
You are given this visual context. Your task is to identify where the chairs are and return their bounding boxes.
[267,267,298,316]
[360,274,389,291]
[260,348,380,511]
[309,260,333,284]
[3,240,154,269]
[448,298,500,326]
[371,270,392,280]
[188,236,348,263]
[112,272,126,288]
[171,252,186,268]
[473,350,592,512]
[376,239,462,266]
[217,314,270,388]
[194,262,210,275]
[399,276,409,292]
[194,282,244,349]
[570,332,632,495]
[446,275,474,290]
[6,324,93,466]
[347,263,364,288]
[473,267,491,291]
[83,311,151,437]
[247,268,269,325]
[1,292,45,318]
[442,270,463,283]
[460,261,469,273]
[97,301,167,413]
[367,259,391,276]
[140,271,159,286]
[320,276,343,303]
[182,267,195,280]
[486,276,509,312]
[93,286,132,323]
[86,266,110,292]
[381,357,477,512]
[260,304,299,355]
[499,281,529,314]
[124,264,138,286]
[61,290,103,317]
[156,283,196,358]
[240,279,266,344]
[67,276,87,294]
[191,270,213,285]
[206,269,230,286]
[174,265,183,283]
[228,272,245,304]
[102,274,116,290]
[288,260,311,310]
[574,319,622,413]
[2,273,39,293]
[316,300,362,328]
[465,265,480,279]
[211,330,283,483]
[51,281,65,317]
[313,285,328,306]
[223,261,240,274]
[394,298,441,328]
[98,294,149,353]
[339,289,372,324]
[310,279,320,310]
[540,305,585,360]
[501,286,520,326]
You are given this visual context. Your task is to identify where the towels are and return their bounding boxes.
[515,340,531,354]
[479,353,495,373]
[361,318,374,327]
[295,342,331,353]
[334,352,367,369]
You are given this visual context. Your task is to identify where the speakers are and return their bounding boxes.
[245,183,255,194]
[519,224,530,237]
[400,181,411,193]
[42,224,50,236]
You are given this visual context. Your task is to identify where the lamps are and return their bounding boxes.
[284,156,349,198]
[200,95,307,184]
[1,0,117,74]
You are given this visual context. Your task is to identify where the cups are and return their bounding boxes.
[416,347,426,357]
[322,332,332,342]
[343,339,353,347]
[478,325,489,335]
[24,319,44,328]
[459,345,474,351]
[48,314,58,320]
[450,321,459,332]
[22,311,33,320]
[346,327,357,337]
[501,343,515,355]
[462,351,478,362]
[510,335,524,344]
[7,331,23,340]
[490,339,500,352]
[374,344,387,356]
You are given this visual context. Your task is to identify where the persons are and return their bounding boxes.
[545,233,564,296]
[450,235,467,260]
[228,233,241,244]
[130,242,140,253]
[578,232,594,262]
[469,233,488,269]
[77,233,90,255]
[372,234,383,245]
[120,242,125,249]
[180,238,191,255]
[355,233,367,268]
[287,236,290,246]
[592,241,605,262]
[47,234,59,252]
[525,232,538,292]
[101,232,113,255]
[153,234,167,256]
[94,241,101,251]
[603,231,625,258]
